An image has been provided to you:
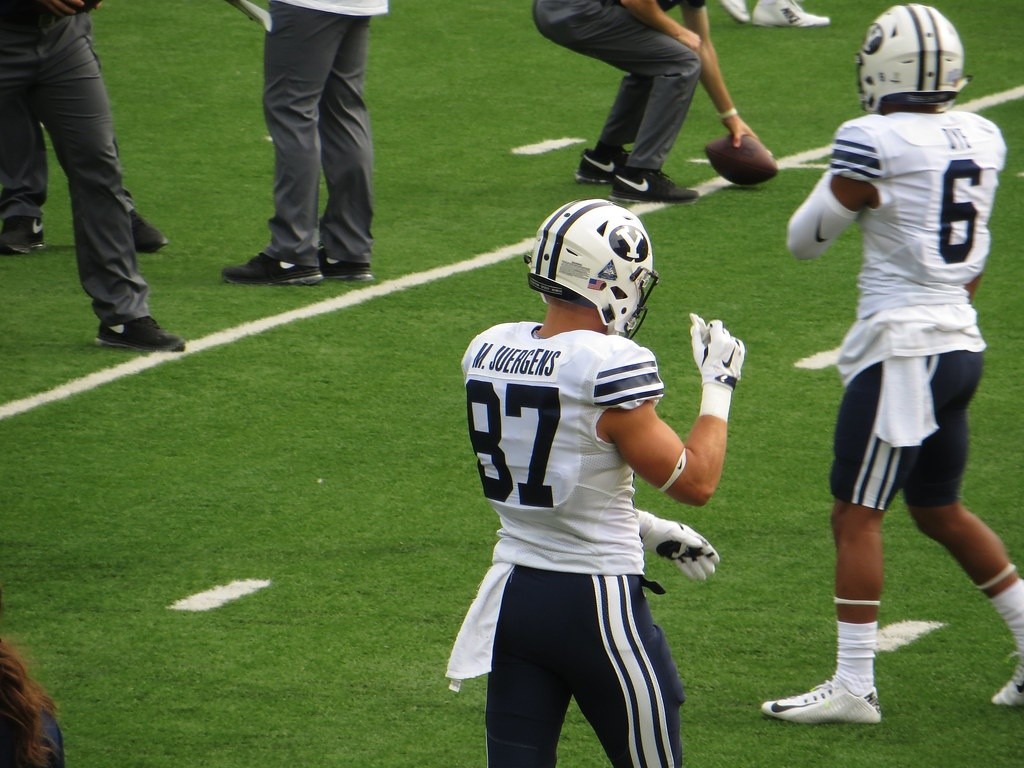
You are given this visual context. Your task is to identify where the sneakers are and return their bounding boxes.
[313,249,377,284]
[749,0,834,28]
[988,668,1024,711]
[0,214,46,254]
[222,252,323,287]
[93,309,189,353]
[573,149,624,186]
[129,207,173,252]
[720,0,750,25]
[608,167,700,207]
[759,678,886,728]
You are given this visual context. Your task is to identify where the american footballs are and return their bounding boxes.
[704,135,779,186]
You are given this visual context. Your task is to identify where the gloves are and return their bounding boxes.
[639,515,723,582]
[688,312,750,390]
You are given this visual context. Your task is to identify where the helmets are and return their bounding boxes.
[854,2,972,107]
[519,197,659,339]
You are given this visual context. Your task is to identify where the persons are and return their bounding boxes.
[762,2,1024,723]
[0,0,186,351]
[0,635,67,768]
[446,196,746,768]
[217,0,391,292]
[530,0,834,203]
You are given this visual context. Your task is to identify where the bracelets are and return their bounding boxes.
[719,107,736,120]
